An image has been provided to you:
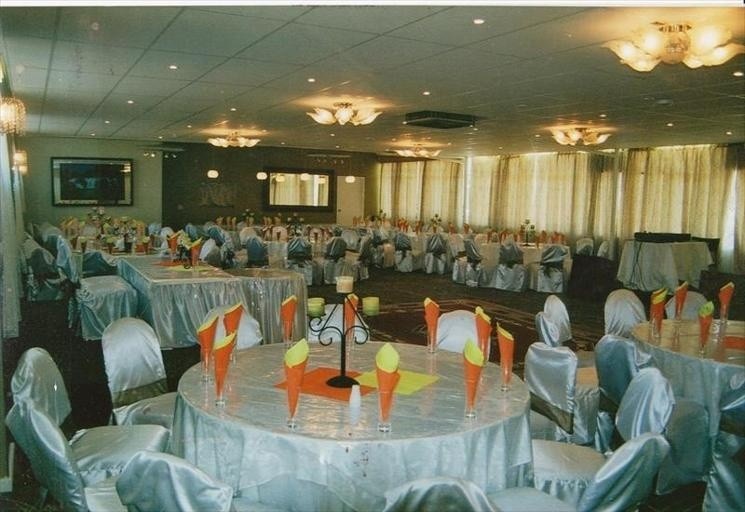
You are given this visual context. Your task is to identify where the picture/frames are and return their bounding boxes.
[50,157,133,206]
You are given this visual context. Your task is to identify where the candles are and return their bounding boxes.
[336,276,353,293]
[362,297,379,315]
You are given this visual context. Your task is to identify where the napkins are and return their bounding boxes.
[282,339,310,417]
[210,330,238,400]
[424,298,440,346]
[474,307,492,355]
[373,343,400,422]
[461,339,486,407]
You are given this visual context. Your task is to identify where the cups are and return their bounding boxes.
[501,358,513,391]
[225,321,491,367]
[284,385,301,428]
[377,389,395,433]
[463,375,482,420]
[200,347,212,383]
[644,302,730,356]
[212,367,228,407]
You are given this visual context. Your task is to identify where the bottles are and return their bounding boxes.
[348,383,362,425]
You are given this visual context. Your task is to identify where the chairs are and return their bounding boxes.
[18,216,346,349]
[102,318,178,430]
[383,478,500,511]
[570,237,611,260]
[435,310,491,360]
[1,347,235,512]
[341,217,573,294]
[304,304,370,343]
[487,288,745,511]
[486,431,671,512]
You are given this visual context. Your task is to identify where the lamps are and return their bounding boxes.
[306,102,382,127]
[394,145,443,158]
[546,126,613,146]
[207,131,260,149]
[0,96,27,137]
[602,23,745,73]
